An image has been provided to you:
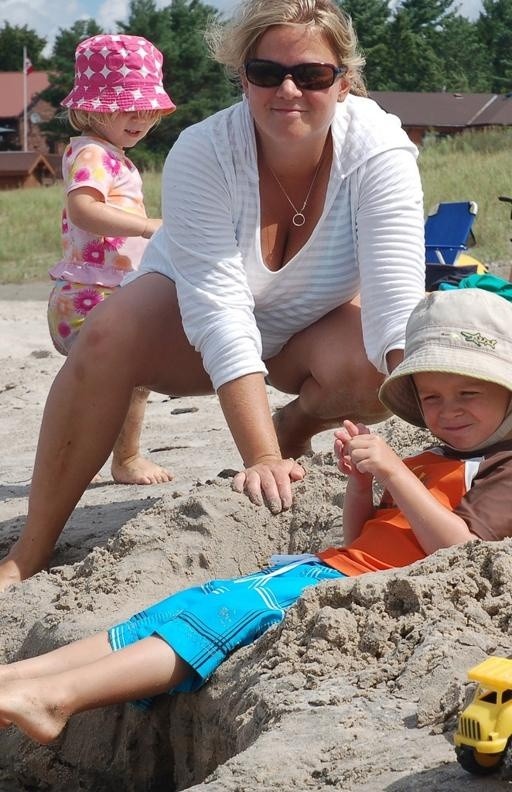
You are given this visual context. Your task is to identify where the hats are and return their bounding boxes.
[60,33,176,113]
[377,288,512,428]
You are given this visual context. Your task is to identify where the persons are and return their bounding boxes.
[0,0,428,591]
[0,290,511,747]
[47,33,176,485]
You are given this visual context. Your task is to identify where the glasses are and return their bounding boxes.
[241,58,343,91]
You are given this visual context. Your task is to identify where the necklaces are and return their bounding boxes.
[257,134,326,227]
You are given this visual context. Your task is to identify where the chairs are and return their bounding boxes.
[423,200,479,266]
